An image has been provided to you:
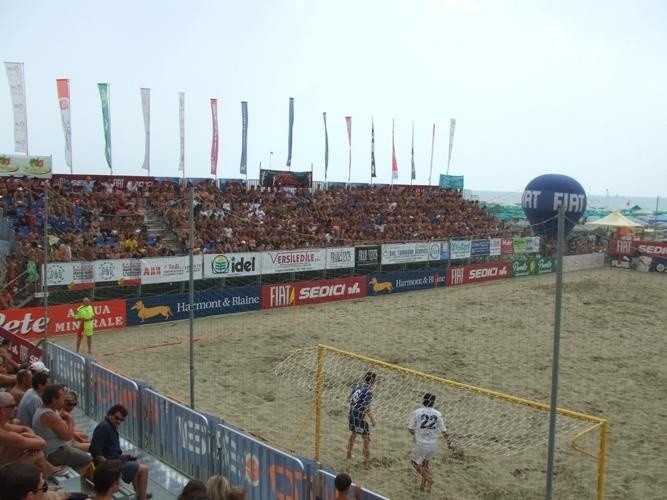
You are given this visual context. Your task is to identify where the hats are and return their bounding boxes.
[28,361,50,375]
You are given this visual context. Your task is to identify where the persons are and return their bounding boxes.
[408,393,454,498]
[540,236,604,259]
[1,334,354,499]
[1,172,533,311]
[347,371,377,464]
[73,298,94,354]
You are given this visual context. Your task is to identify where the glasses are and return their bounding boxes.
[111,413,124,422]
[31,480,49,494]
[64,401,77,407]
[2,402,18,409]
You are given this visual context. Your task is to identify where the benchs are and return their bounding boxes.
[84,477,136,500]
